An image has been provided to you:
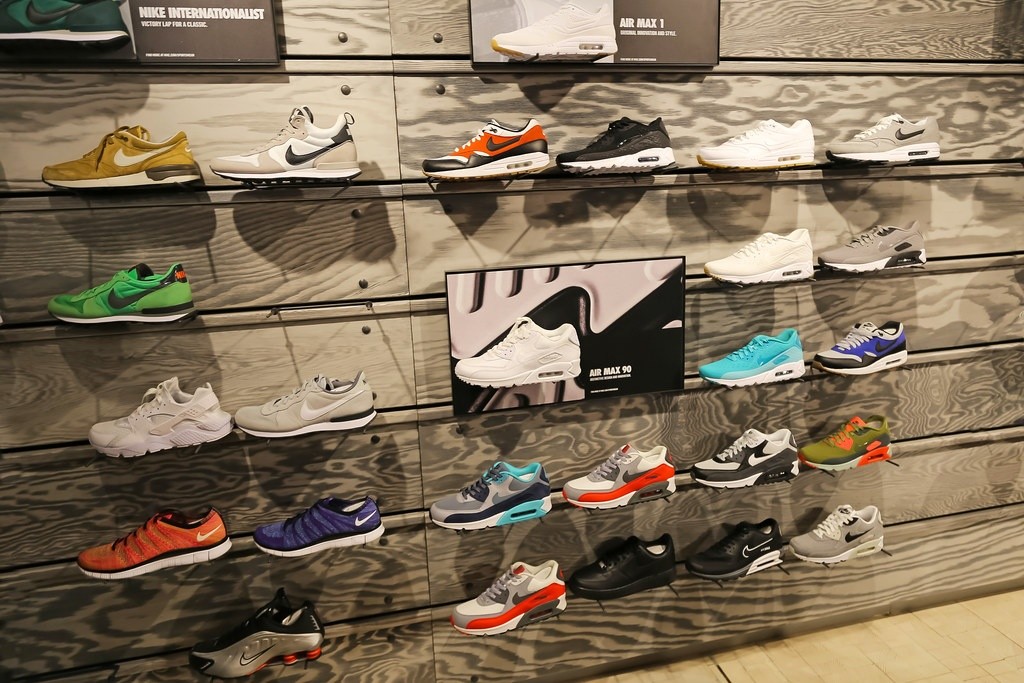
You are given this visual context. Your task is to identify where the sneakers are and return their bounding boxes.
[490,3,618,59]
[42,125,201,189]
[209,104,362,184]
[824,113,942,166]
[429,461,552,531]
[74,506,232,580]
[695,118,816,171]
[818,219,927,273]
[788,504,884,564]
[88,375,234,458]
[797,414,893,472]
[47,262,195,324]
[187,589,325,678]
[555,116,676,175]
[686,517,784,582]
[702,227,815,285]
[699,328,806,388]
[810,320,908,375]
[252,495,385,557]
[421,118,551,181]
[455,316,581,388]
[449,559,567,637]
[234,370,376,437]
[571,533,677,600]
[0,0,132,52]
[689,428,799,489]
[562,443,676,509]
